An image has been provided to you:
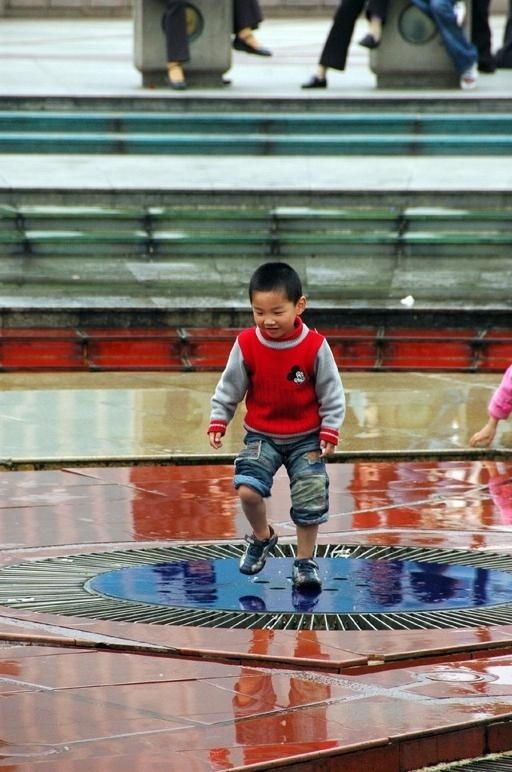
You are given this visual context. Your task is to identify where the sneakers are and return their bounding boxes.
[238,524,278,576]
[290,555,322,591]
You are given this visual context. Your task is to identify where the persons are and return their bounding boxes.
[480,459,512,530]
[471,0,511,73]
[184,561,218,606]
[469,361,511,449]
[358,558,405,604]
[410,1,475,89]
[299,1,390,90]
[207,592,341,772]
[205,262,348,590]
[161,0,273,90]
